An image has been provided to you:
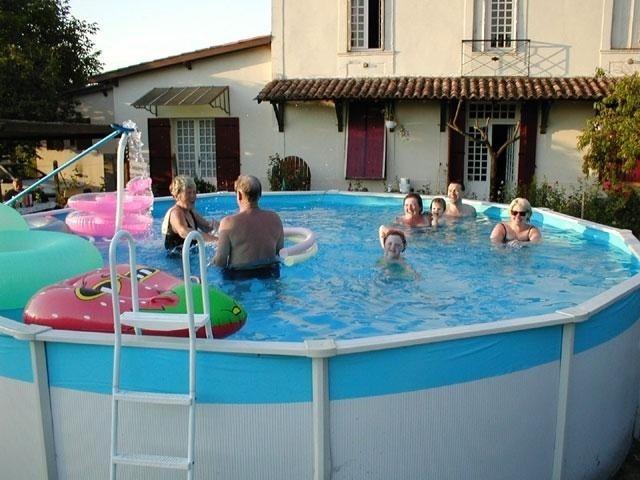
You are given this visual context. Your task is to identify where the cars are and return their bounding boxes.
[0,159,84,199]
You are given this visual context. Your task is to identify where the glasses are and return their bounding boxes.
[511,211,529,216]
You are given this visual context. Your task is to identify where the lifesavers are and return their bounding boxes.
[0,205,104,310]
[19,213,69,235]
[64,191,154,237]
[22,263,246,339]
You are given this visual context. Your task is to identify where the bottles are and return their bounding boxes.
[388,175,410,193]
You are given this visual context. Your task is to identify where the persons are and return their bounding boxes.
[3,177,27,208]
[376,229,415,275]
[444,180,476,217]
[209,174,284,280]
[490,197,541,250]
[424,198,447,228]
[393,192,431,226]
[162,175,219,258]
